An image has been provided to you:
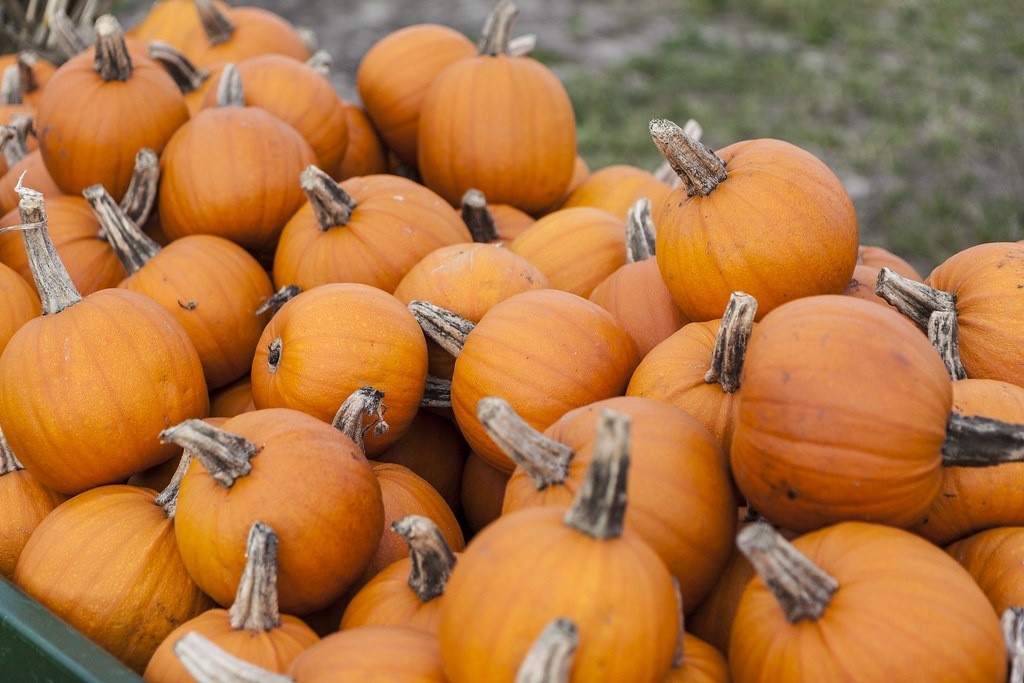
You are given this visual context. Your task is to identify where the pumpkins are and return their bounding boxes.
[0,0,1024,683]
